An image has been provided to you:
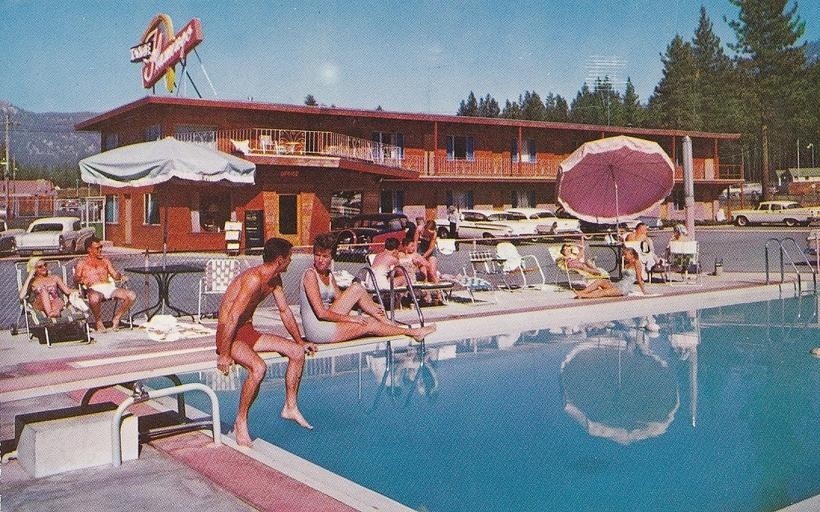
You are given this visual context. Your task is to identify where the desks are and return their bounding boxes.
[122,263,205,325]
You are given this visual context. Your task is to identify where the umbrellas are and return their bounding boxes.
[79,136,257,269]
[554,136,675,242]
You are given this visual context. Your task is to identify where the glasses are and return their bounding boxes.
[37,262,48,268]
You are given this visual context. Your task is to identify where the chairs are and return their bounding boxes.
[495,243,547,290]
[9,260,90,347]
[198,258,242,324]
[365,254,453,307]
[623,233,664,283]
[62,256,133,331]
[668,240,702,282]
[549,244,610,289]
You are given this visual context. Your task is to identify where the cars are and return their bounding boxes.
[505,209,581,243]
[328,213,420,263]
[723,182,762,197]
[0,219,25,253]
[11,217,96,258]
[731,200,820,227]
[433,210,538,247]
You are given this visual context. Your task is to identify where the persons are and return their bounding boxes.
[72,237,136,332]
[557,244,601,275]
[368,239,408,310]
[216,239,318,445]
[626,233,663,265]
[401,237,432,304]
[447,205,460,252]
[751,191,762,208]
[573,247,654,298]
[21,257,82,317]
[633,224,654,282]
[666,224,696,264]
[413,220,437,272]
[300,231,436,344]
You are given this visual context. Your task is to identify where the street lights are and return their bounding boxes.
[807,143,814,169]
